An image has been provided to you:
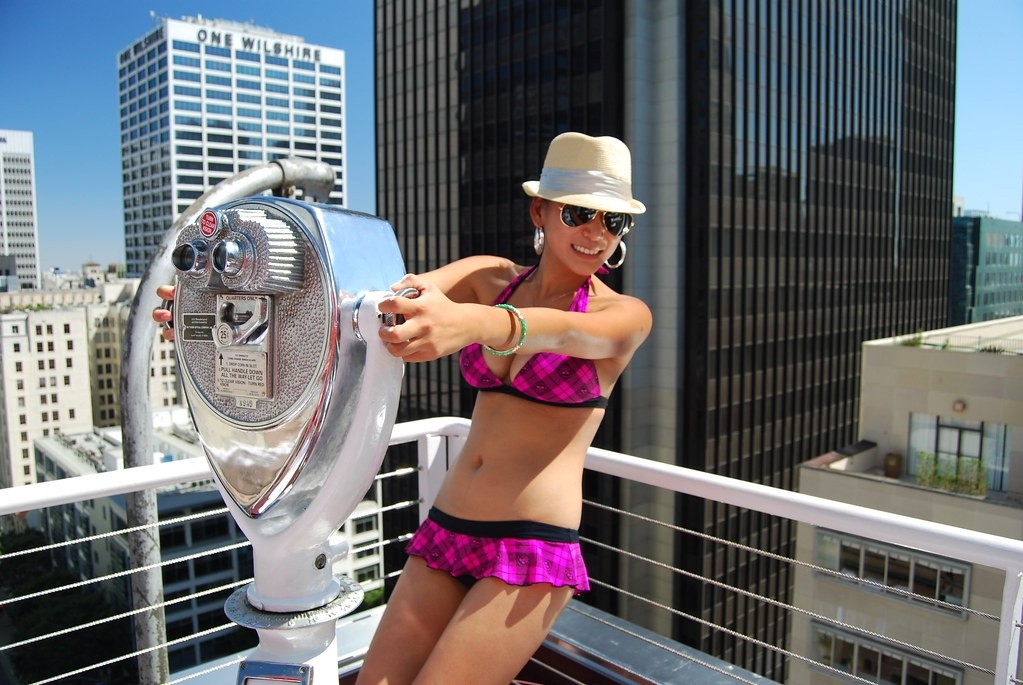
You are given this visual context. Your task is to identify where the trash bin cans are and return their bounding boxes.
[885,453,902,478]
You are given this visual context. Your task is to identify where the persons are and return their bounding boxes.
[154,132,652,685]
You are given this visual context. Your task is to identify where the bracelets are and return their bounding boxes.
[483,304,527,356]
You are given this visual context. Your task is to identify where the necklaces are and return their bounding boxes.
[540,291,573,306]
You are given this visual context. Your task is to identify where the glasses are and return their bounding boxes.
[549,202,625,237]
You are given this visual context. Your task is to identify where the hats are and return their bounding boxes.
[522,132,647,213]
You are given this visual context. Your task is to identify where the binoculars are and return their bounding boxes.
[163,195,419,685]
